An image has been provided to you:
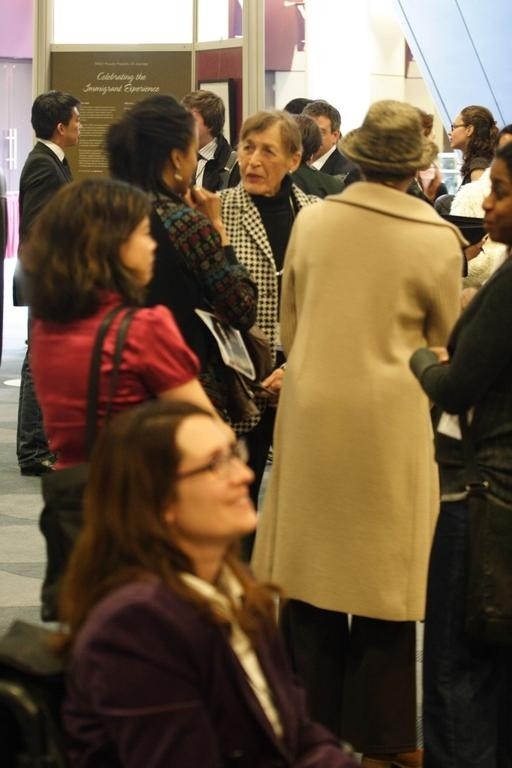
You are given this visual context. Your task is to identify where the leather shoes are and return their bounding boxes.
[21,461,52,477]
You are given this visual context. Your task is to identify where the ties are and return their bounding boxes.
[63,156,73,183]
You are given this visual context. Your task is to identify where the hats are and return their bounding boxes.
[335,99,440,177]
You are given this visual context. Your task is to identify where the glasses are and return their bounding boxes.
[449,123,468,131]
[175,437,252,483]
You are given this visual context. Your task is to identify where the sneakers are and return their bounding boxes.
[356,744,426,768]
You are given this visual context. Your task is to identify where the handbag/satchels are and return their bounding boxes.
[37,464,94,622]
[460,479,512,650]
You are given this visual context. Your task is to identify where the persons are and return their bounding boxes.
[408,138,511,767]
[249,94,464,767]
[58,397,359,767]
